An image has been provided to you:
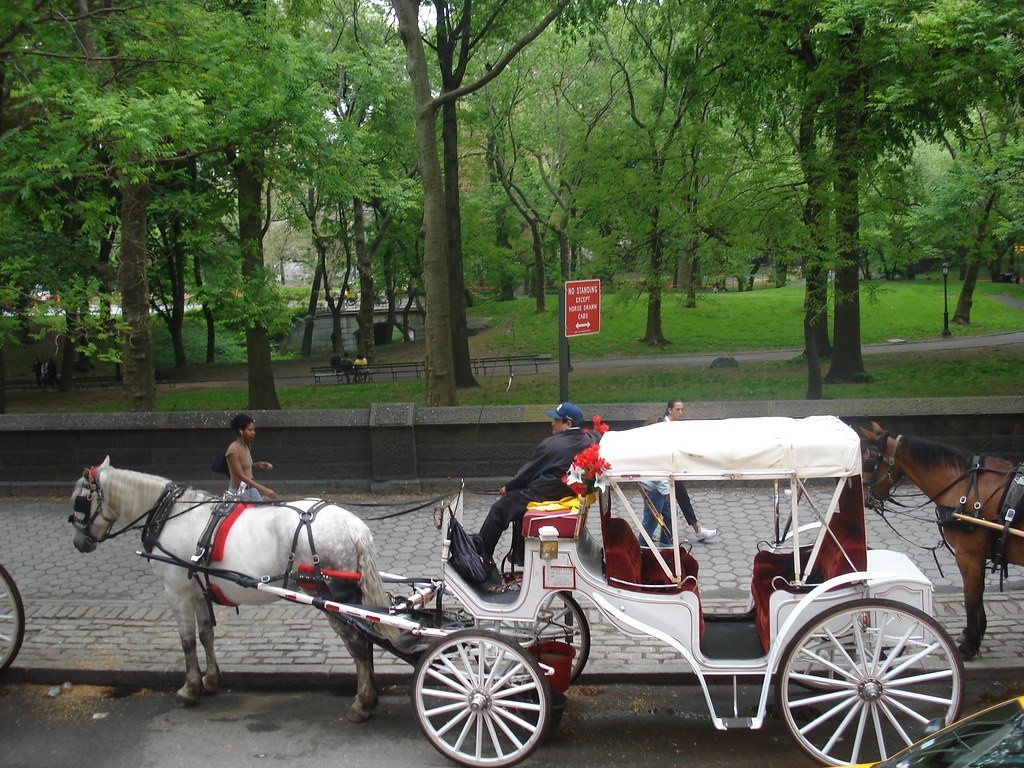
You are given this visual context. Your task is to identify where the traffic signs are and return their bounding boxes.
[564,278,600,338]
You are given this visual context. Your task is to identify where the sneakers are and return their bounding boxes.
[694,525,717,542]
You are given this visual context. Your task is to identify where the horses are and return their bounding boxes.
[68,456,403,722]
[858,422,1024,662]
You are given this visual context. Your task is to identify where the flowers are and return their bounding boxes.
[581,415,608,436]
[562,442,612,500]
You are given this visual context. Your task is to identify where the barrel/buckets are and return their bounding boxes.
[514,689,570,739]
[526,620,576,694]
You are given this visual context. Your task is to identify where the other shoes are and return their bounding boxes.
[660,542,673,547]
[507,552,524,566]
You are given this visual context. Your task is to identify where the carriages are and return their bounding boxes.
[66,413,966,768]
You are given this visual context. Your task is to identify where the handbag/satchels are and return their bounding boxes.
[446,505,492,585]
[210,448,229,475]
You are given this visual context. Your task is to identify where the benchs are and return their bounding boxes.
[521,497,584,538]
[604,517,705,639]
[312,353,553,386]
[751,512,867,654]
[2,371,178,394]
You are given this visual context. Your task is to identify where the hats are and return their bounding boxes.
[545,402,583,426]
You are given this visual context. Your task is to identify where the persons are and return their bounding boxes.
[329,352,342,382]
[353,352,367,383]
[663,399,716,541]
[638,413,673,548]
[226,414,278,502]
[477,402,583,566]
[40,356,49,388]
[347,287,360,302]
[43,355,60,389]
[33,358,42,389]
[340,352,357,384]
